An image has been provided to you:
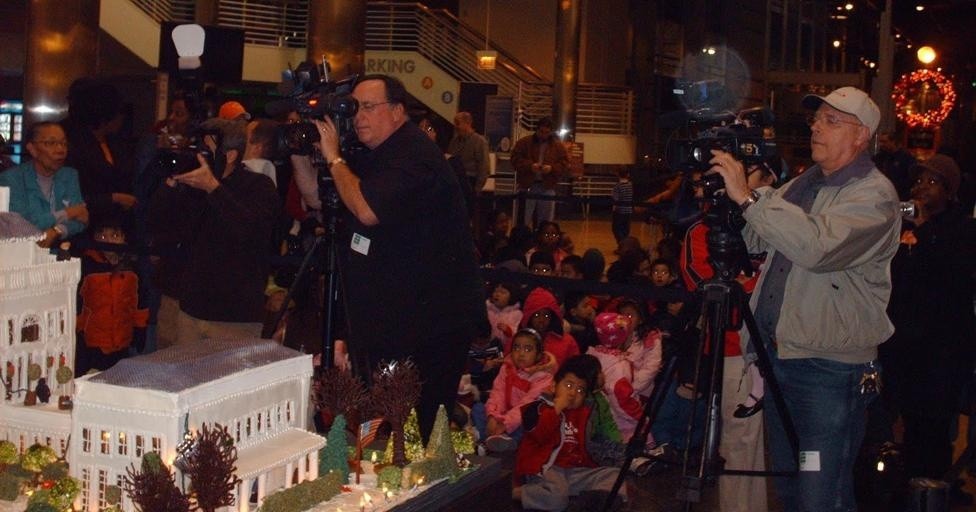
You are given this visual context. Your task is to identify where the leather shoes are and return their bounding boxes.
[733,393,764,419]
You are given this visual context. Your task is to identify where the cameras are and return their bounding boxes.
[897,200,917,220]
[165,141,214,177]
[282,58,360,155]
[687,104,765,177]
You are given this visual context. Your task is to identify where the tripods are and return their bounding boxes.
[267,160,374,371]
[608,202,810,512]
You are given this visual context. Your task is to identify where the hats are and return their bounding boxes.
[799,86,882,138]
[908,154,960,193]
[220,101,251,121]
[594,311,632,349]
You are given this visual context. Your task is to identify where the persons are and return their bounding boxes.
[469,330,558,454]
[459,211,604,361]
[137,86,320,341]
[732,270,764,418]
[509,117,571,227]
[612,166,636,252]
[681,157,795,512]
[509,356,634,512]
[594,236,687,474]
[900,152,976,505]
[446,111,491,196]
[414,114,475,219]
[0,121,89,255]
[311,74,490,453]
[26,79,148,353]
[76,210,158,371]
[706,88,902,512]
[874,130,922,201]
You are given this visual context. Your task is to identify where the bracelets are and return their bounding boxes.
[53,225,63,234]
[326,155,346,171]
[739,190,761,213]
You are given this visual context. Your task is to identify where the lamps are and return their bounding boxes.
[476,0,498,70]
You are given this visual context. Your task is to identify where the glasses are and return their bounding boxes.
[32,135,68,150]
[358,100,394,112]
[419,126,436,135]
[806,113,865,130]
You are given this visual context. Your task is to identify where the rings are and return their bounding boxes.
[719,159,730,168]
[322,127,329,133]
